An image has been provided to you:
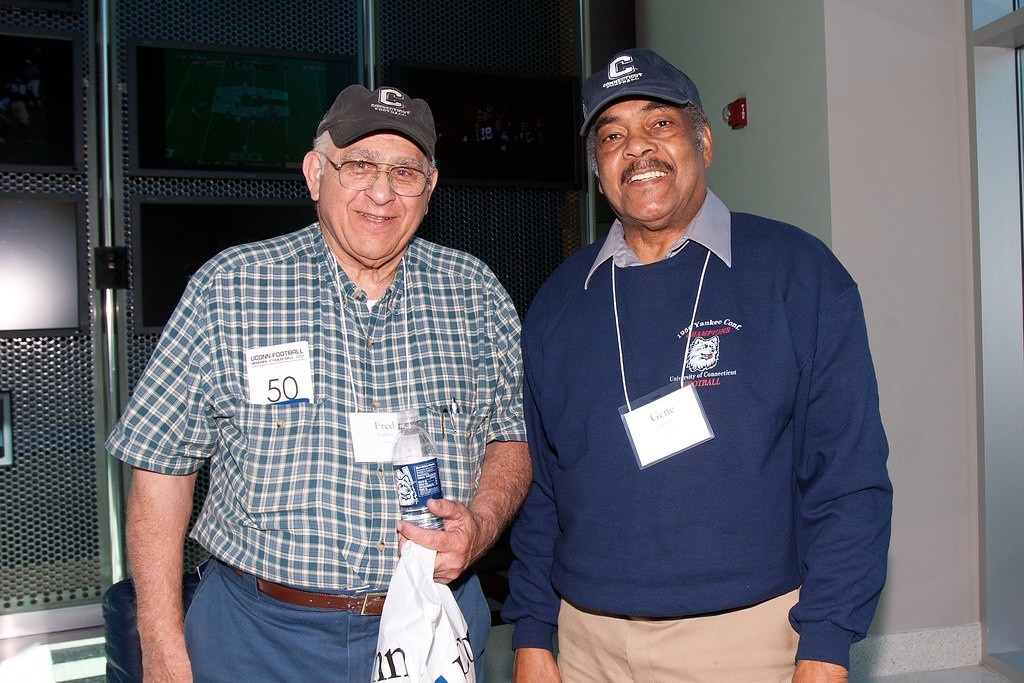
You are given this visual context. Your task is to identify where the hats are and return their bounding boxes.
[580,49,703,137]
[316,85,437,162]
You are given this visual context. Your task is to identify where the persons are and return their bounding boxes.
[500,53,893,683]
[106,83,532,683]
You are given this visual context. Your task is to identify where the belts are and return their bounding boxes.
[229,564,387,618]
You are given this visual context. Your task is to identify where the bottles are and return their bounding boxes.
[392,409,444,531]
